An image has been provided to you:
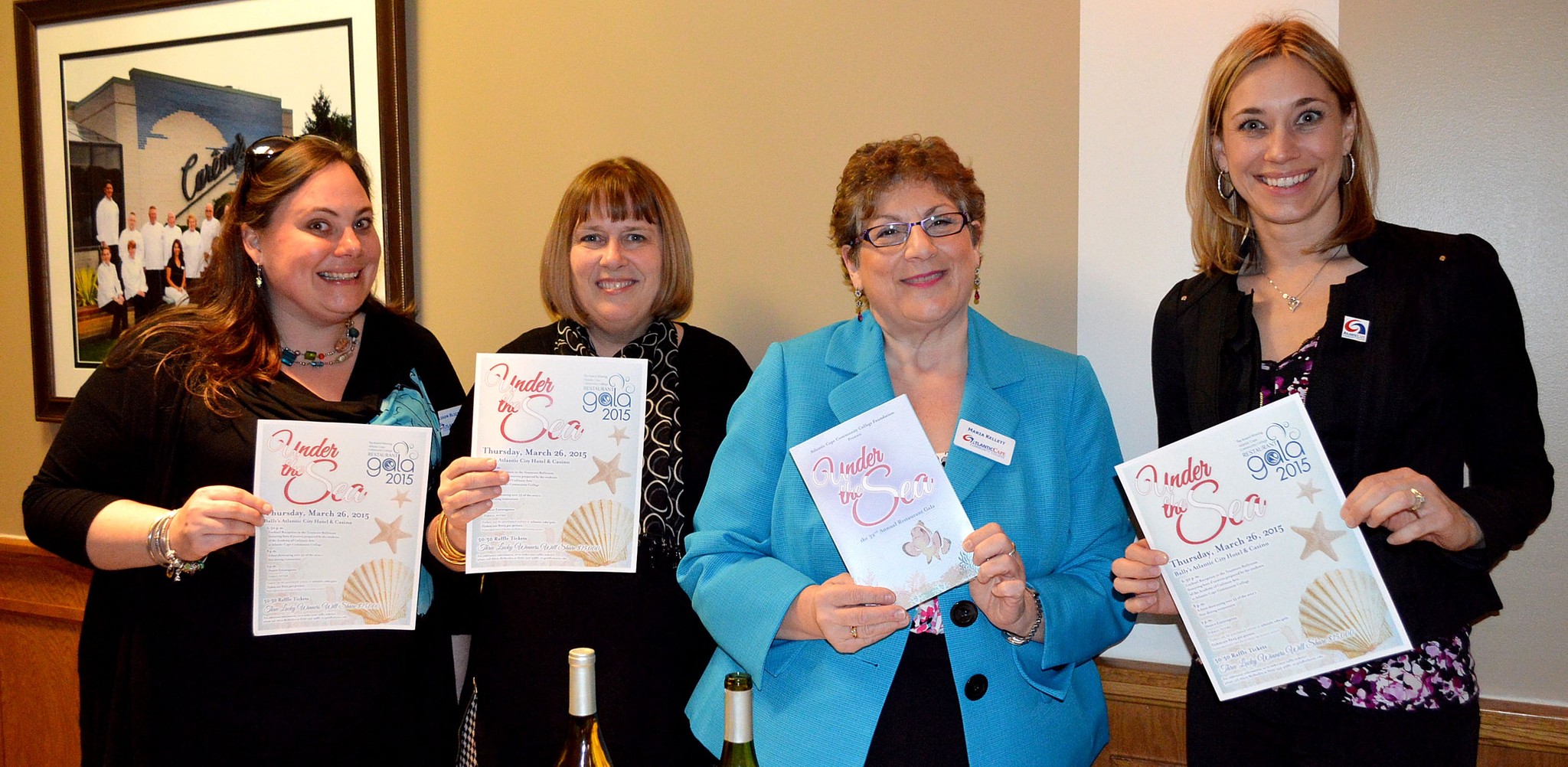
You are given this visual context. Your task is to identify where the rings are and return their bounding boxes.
[1411,488,1426,510]
[850,626,857,638]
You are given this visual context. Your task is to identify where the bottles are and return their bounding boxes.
[715,673,759,767]
[554,648,613,767]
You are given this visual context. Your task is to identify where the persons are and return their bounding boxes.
[95,180,121,280]
[182,213,205,289]
[97,247,129,339]
[675,133,1136,767]
[121,240,149,320]
[118,212,145,264]
[140,205,169,310]
[164,210,182,258]
[423,155,754,767]
[1110,19,1555,767]
[200,203,222,270]
[21,134,468,767]
[164,238,189,306]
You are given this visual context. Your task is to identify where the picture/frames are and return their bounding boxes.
[12,1,414,422]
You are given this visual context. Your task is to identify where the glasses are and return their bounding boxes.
[240,134,308,206]
[848,211,969,246]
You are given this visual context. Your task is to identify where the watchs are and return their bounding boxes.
[1001,586,1043,645]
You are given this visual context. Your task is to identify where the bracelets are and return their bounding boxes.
[146,508,208,581]
[435,511,466,565]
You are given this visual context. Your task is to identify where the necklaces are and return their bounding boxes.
[281,319,359,367]
[1259,244,1344,313]
